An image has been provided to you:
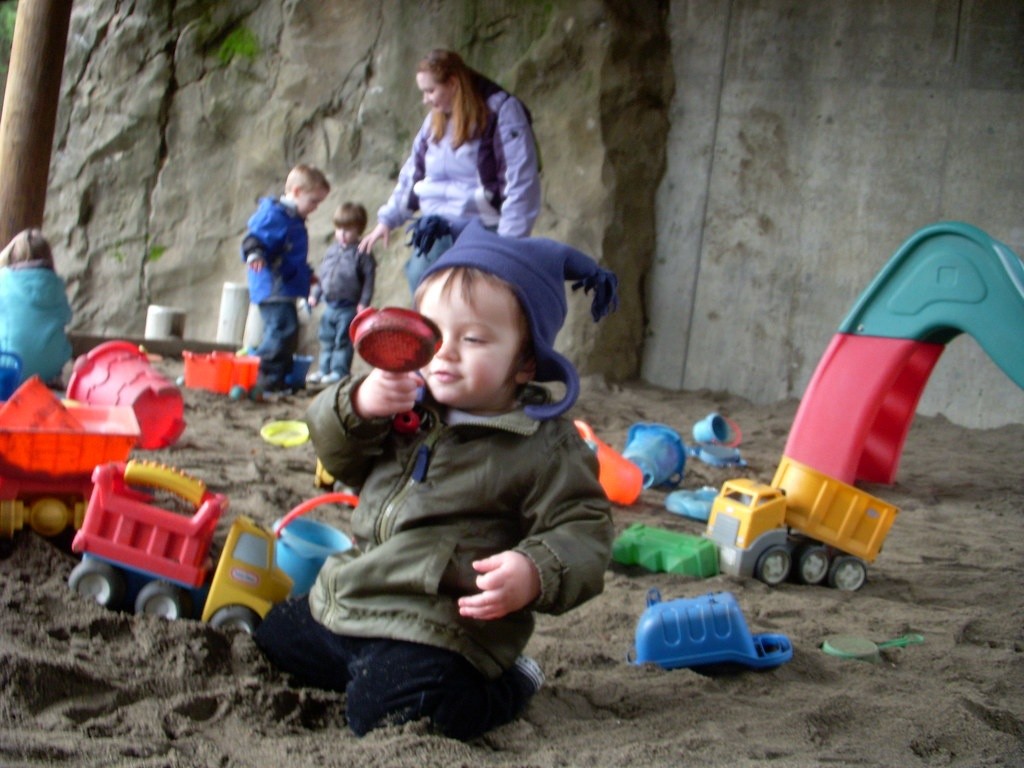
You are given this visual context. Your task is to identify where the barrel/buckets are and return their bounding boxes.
[691,413,742,447]
[65,338,184,451]
[621,422,686,489]
[276,494,360,599]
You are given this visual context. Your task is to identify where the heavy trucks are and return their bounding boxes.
[67,459,295,642]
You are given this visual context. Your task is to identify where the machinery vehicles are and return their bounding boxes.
[701,218,1023,590]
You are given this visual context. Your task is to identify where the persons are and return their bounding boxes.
[357,49,541,307]
[251,220,620,742]
[307,202,376,385]
[0,227,73,390]
[240,164,331,402]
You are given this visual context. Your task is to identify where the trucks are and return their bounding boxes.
[0,400,142,538]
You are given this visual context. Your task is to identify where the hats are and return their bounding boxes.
[405,212,617,419]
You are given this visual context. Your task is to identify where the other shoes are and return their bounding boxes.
[321,371,346,382]
[306,371,325,381]
[248,381,291,402]
[503,657,544,704]
